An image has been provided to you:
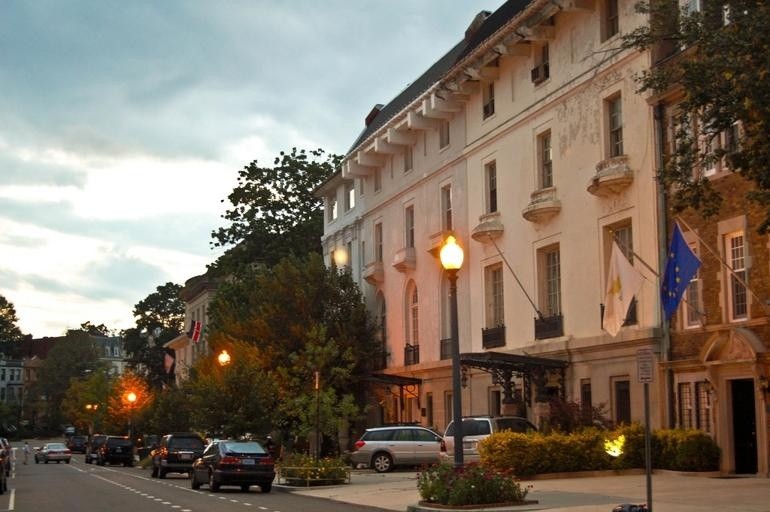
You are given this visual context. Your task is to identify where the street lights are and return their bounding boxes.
[438,235,467,470]
[217,349,232,437]
[127,393,138,439]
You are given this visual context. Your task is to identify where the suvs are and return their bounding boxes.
[97,436,135,467]
[65,435,86,454]
[150,431,207,479]
[84,433,109,463]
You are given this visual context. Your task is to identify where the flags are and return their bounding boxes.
[602,243,643,337]
[164,354,174,373]
[186,319,203,344]
[660,222,702,321]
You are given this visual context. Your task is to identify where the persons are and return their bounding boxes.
[22,441,32,465]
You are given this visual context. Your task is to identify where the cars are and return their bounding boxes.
[0,437,11,494]
[64,426,76,436]
[34,443,72,464]
[190,439,276,495]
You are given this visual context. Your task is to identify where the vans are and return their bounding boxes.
[344,427,444,472]
[137,435,160,459]
[439,416,537,468]
